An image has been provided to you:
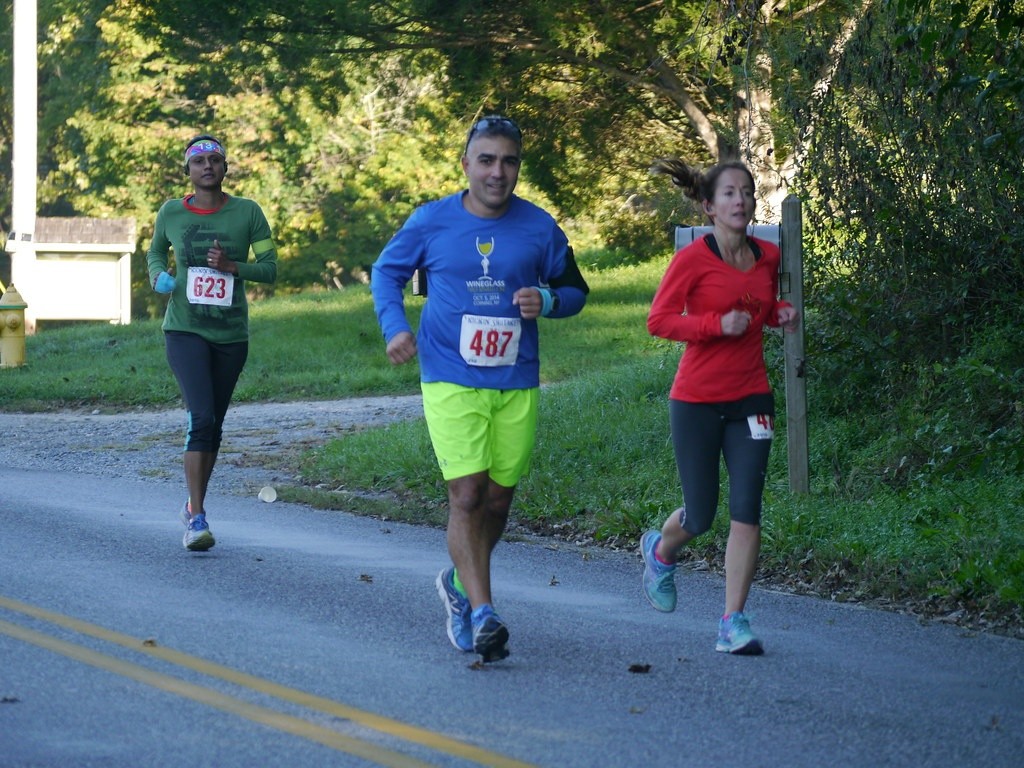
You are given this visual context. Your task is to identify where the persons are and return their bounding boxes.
[640,159,802,652]
[147,136,277,552]
[372,114,591,665]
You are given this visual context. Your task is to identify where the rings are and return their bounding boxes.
[209,258,212,262]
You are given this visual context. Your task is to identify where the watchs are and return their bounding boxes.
[545,288,560,318]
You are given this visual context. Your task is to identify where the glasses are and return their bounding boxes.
[465,118,523,155]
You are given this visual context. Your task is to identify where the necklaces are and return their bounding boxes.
[193,196,196,207]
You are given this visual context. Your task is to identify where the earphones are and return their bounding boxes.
[706,206,713,212]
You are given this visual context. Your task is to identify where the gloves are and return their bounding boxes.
[155,272,176,294]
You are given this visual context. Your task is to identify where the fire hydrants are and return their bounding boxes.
[0,282,29,370]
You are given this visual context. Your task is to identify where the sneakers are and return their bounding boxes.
[179,503,207,525]
[640,530,677,613]
[435,566,472,652]
[470,604,510,665]
[716,610,763,653]
[182,515,215,551]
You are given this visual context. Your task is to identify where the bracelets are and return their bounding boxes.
[531,286,552,316]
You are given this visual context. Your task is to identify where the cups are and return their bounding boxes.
[258,486,277,503]
